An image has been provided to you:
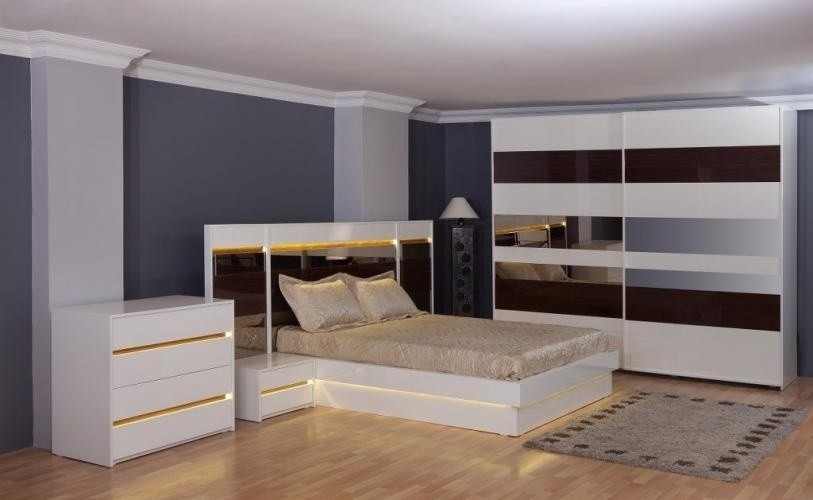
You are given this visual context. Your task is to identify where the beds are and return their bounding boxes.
[275,311,619,438]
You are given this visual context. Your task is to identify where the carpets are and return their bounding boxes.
[526,389,810,483]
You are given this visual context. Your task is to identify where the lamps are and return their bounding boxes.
[439,197,483,232]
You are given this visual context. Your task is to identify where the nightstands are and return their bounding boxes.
[236,353,317,423]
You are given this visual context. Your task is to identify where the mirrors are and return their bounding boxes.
[494,214,622,287]
[214,239,433,359]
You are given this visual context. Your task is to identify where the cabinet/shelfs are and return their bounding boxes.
[52,295,237,469]
